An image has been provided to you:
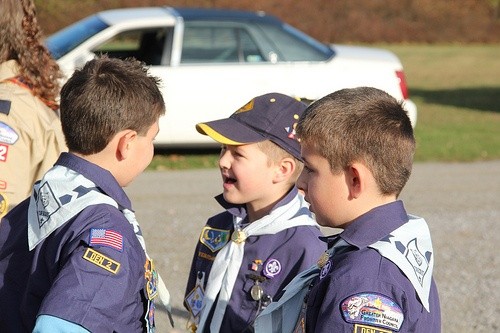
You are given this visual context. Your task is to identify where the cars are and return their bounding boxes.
[41,6,416,150]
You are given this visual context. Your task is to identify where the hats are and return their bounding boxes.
[195,93,308,163]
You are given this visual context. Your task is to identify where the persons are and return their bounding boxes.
[0,0,69,219]
[0,54,174,333]
[182,93,329,333]
[291,87,441,333]
